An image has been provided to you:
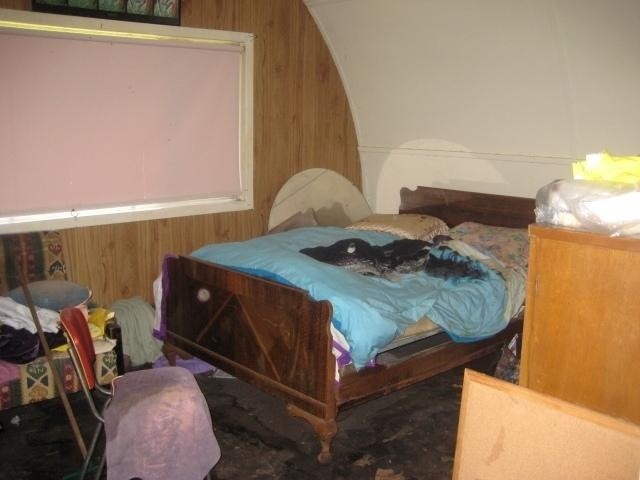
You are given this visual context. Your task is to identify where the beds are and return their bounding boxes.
[0,311,123,411]
[161,185,544,463]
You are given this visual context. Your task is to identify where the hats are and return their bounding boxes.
[329,238,382,276]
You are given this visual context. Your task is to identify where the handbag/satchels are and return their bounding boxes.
[493,345,520,385]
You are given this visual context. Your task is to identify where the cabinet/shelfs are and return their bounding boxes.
[521,222,638,426]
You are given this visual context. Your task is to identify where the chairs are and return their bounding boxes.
[60,307,219,480]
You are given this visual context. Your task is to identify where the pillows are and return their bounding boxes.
[342,213,447,242]
[443,221,526,265]
